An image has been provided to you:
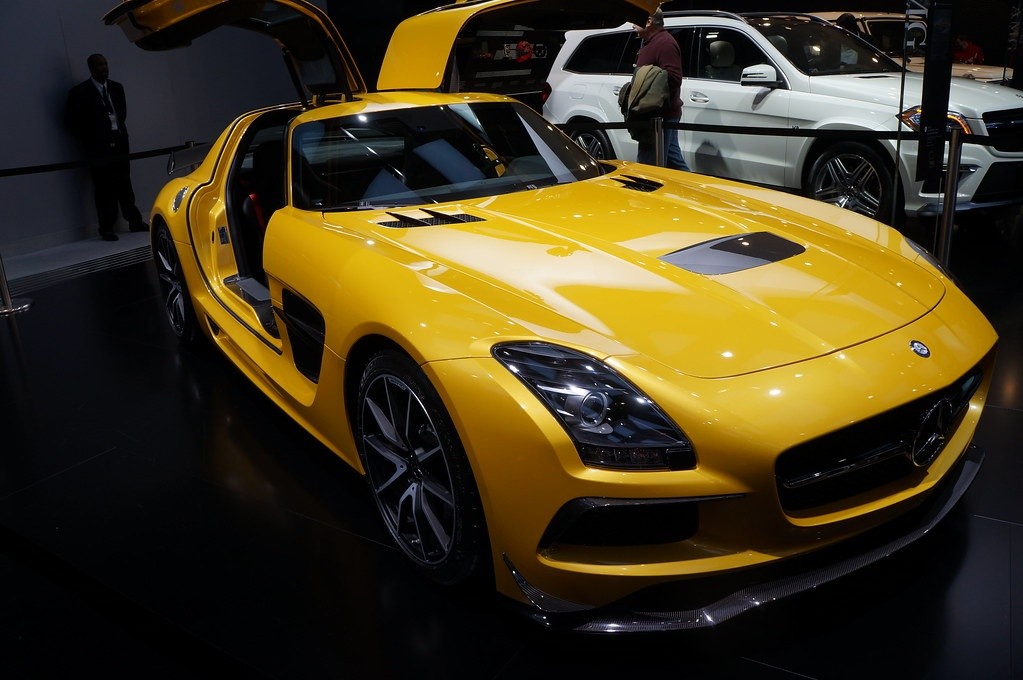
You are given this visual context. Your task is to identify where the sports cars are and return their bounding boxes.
[98,0,1002,646]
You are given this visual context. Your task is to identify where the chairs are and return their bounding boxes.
[704,40,739,80]
[250,139,326,247]
[403,126,480,187]
[767,35,788,58]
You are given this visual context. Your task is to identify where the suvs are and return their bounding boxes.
[542,7,1023,236]
[802,12,1016,87]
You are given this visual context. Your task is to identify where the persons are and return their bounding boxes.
[814,13,880,72]
[619,7,690,172]
[952,34,986,65]
[67,53,149,241]
